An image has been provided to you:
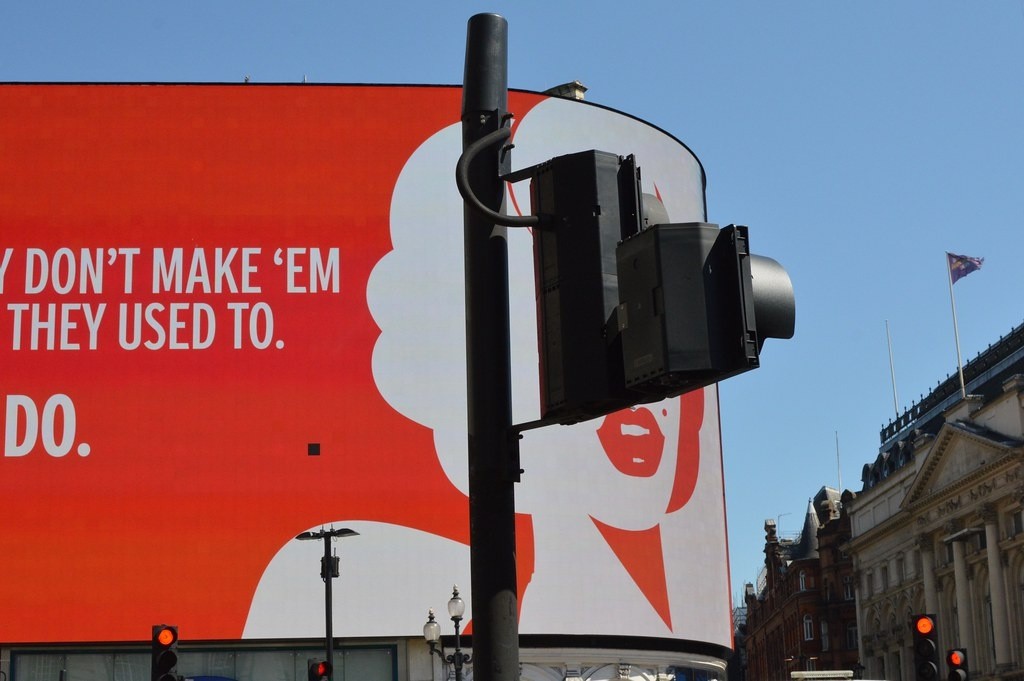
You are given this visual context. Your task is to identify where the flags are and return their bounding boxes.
[948,253,984,285]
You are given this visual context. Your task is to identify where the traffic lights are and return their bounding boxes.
[945,646,969,681]
[525,147,670,426]
[614,220,797,400]
[150,624,180,681]
[307,657,333,681]
[909,612,941,681]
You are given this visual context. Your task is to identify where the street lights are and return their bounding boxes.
[294,523,360,681]
[783,655,818,671]
[423,582,474,681]
